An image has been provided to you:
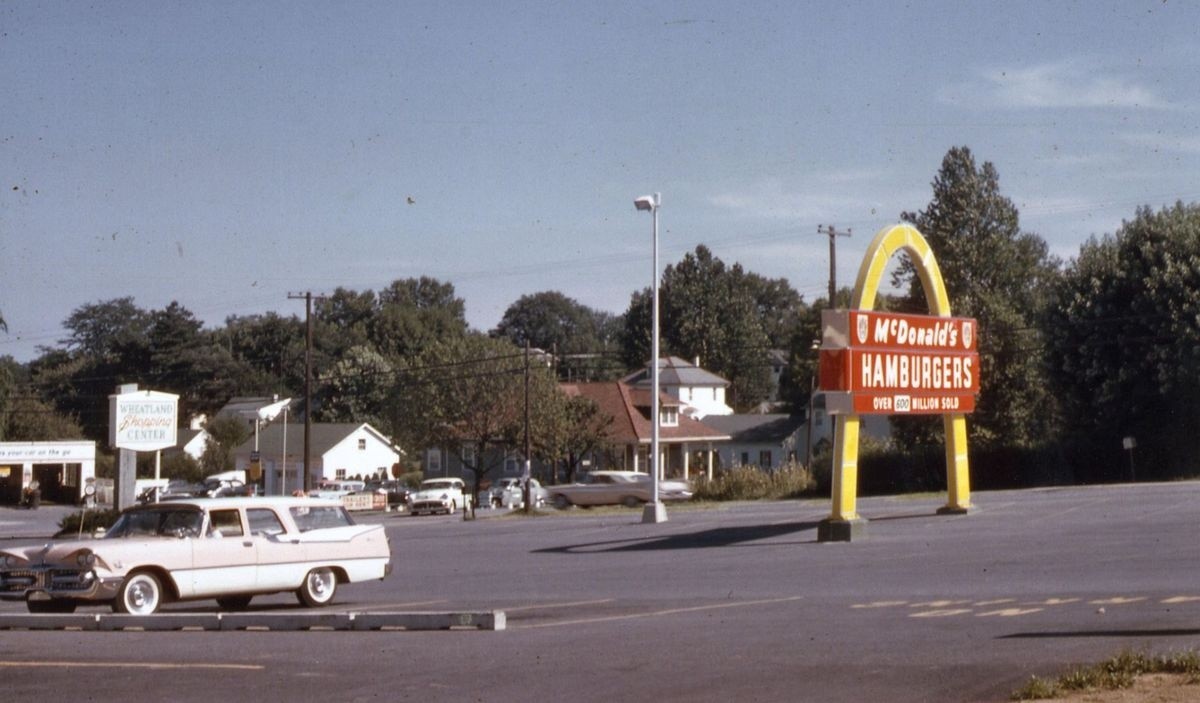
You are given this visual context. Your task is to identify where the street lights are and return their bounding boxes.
[633,192,670,524]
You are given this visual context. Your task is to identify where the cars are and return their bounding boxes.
[195,479,249,498]
[309,478,408,509]
[0,496,393,617]
[405,477,475,516]
[476,475,544,509]
[540,468,694,507]
